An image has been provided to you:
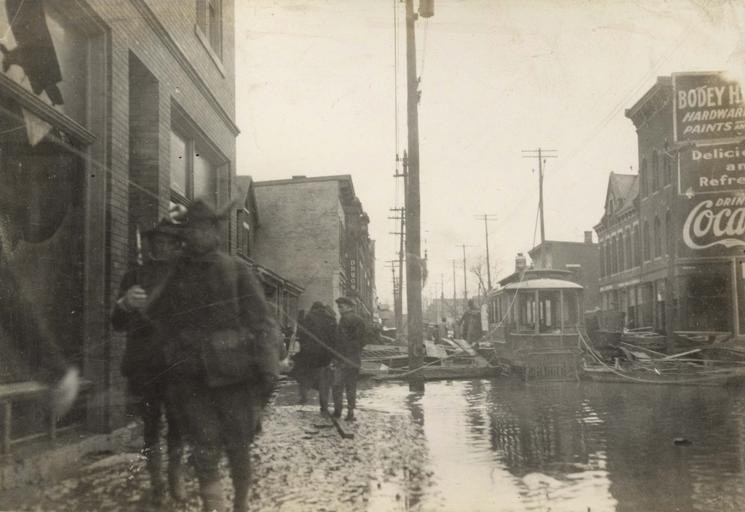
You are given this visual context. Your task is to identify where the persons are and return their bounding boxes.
[329,297,369,420]
[432,325,439,345]
[111,216,191,505]
[456,299,482,343]
[437,317,449,340]
[295,299,333,418]
[150,198,289,512]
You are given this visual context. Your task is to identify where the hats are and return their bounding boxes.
[335,297,355,306]
[142,218,183,247]
[175,200,218,220]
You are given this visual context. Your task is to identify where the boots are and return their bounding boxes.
[143,444,251,511]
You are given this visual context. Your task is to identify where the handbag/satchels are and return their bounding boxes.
[198,329,254,387]
